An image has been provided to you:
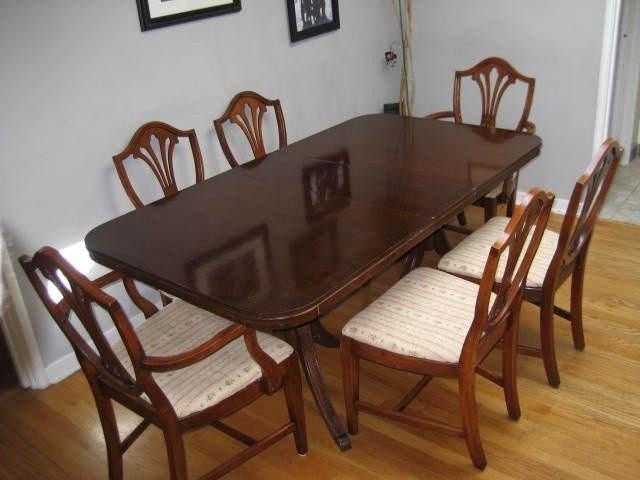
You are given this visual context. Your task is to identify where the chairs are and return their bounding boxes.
[336,185,556,472]
[424,56,536,236]
[111,120,205,308]
[212,90,287,168]
[435,136,625,389]
[16,245,310,480]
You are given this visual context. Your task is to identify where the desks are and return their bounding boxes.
[83,112,543,453]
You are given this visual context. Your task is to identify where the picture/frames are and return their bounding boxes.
[136,0,242,32]
[183,220,277,306]
[300,146,352,227]
[287,0,341,42]
